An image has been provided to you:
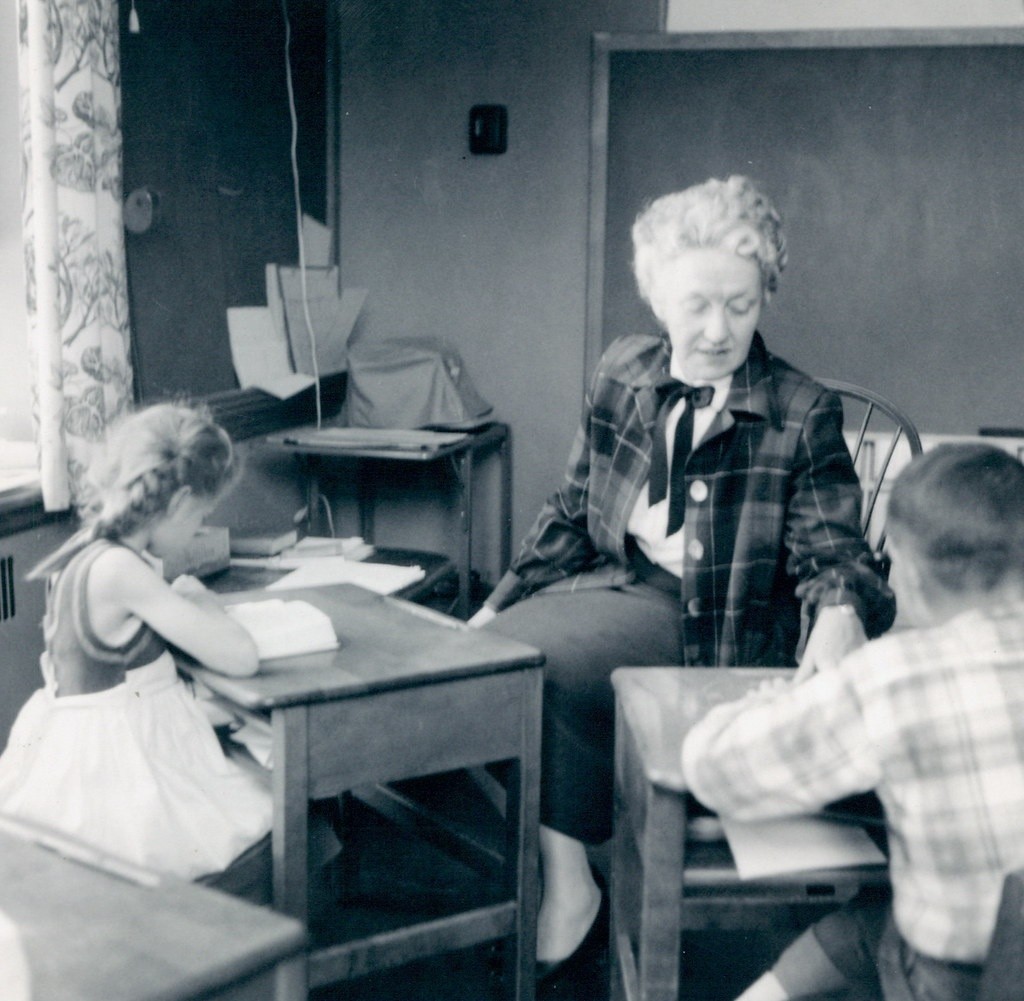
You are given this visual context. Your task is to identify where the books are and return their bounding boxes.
[229,529,425,596]
[224,599,340,657]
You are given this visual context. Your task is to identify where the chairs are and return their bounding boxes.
[813,377,924,579]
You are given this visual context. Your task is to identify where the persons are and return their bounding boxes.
[465,175,895,988]
[0,402,388,953]
[683,440,1024,1001]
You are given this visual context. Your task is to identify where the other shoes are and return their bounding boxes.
[492,864,610,1001]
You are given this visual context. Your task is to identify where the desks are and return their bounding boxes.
[0,810,311,1001]
[252,425,514,614]
[607,664,889,1001]
[172,582,547,1001]
